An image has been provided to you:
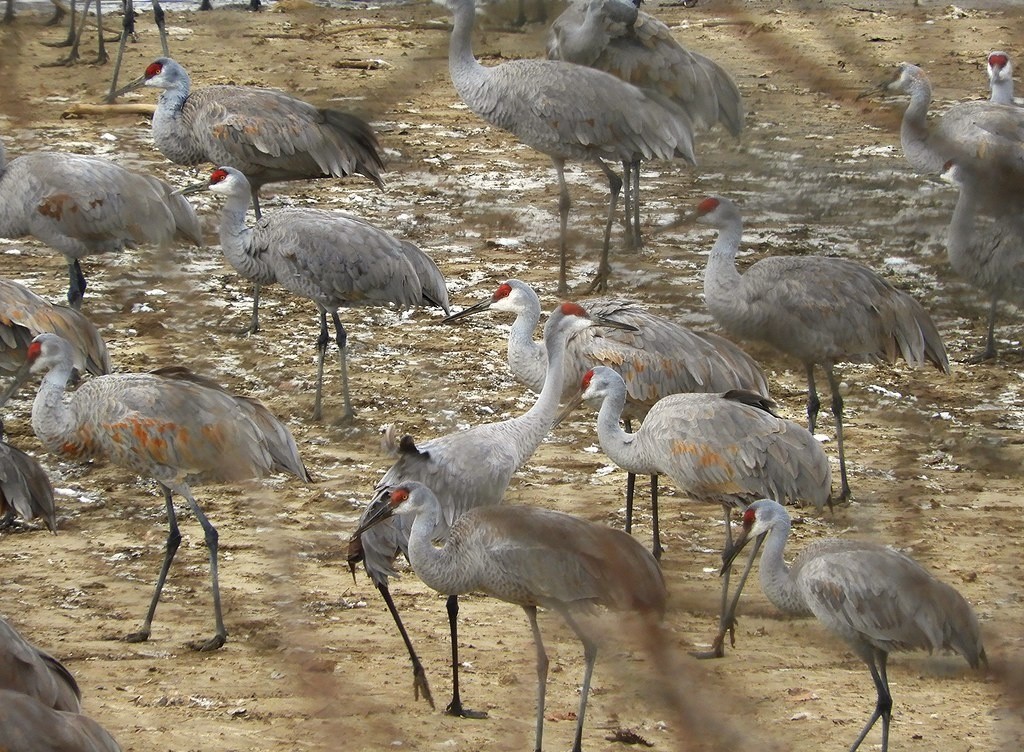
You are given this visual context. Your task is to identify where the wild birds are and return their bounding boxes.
[0,618,121,752]
[0,0,171,103]
[719,497,991,751]
[548,366,832,660]
[0,332,316,651]
[441,278,770,561]
[0,275,111,409]
[348,479,669,752]
[167,166,452,425]
[100,57,389,337]
[436,0,746,301]
[852,51,1024,367]
[0,139,204,311]
[0,440,58,537]
[357,300,640,720]
[650,195,952,515]
